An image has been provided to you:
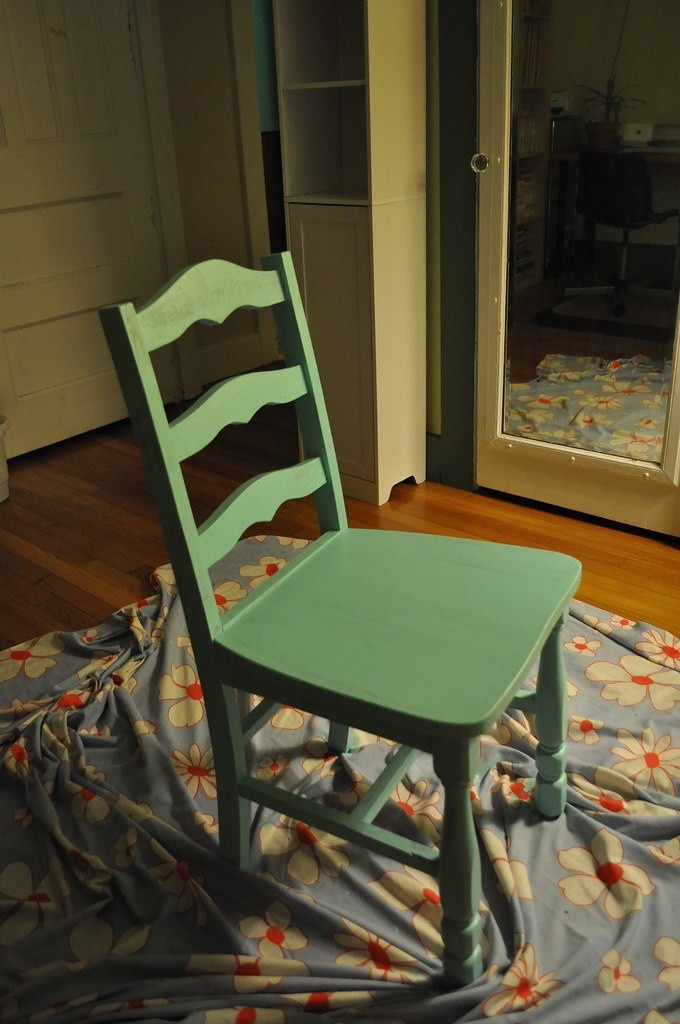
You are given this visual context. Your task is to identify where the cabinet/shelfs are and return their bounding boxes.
[509,0,556,294]
[273,0,427,506]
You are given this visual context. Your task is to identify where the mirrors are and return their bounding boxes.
[485,0,680,488]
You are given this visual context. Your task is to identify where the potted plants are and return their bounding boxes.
[574,69,647,145]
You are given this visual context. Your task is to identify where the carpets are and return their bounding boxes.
[1,536,680,1024]
[505,352,673,464]
[532,277,678,341]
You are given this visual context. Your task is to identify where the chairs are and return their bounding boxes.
[548,142,680,317]
[101,247,583,986]
